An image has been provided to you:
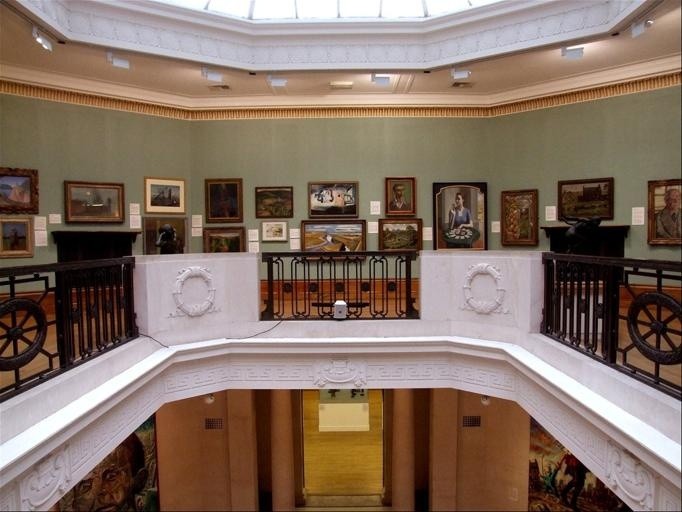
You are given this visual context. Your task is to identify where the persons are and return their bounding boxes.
[656,189,682,238]
[73,432,149,512]
[447,192,473,248]
[390,183,410,211]
[562,451,591,512]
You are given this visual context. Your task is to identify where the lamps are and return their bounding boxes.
[448,64,473,81]
[101,46,132,70]
[27,23,56,54]
[479,394,491,405]
[263,72,289,90]
[625,15,655,40]
[205,393,216,403]
[197,63,225,86]
[367,70,393,89]
[559,42,586,62]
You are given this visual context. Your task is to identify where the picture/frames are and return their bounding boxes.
[203,177,245,224]
[499,188,540,248]
[1,165,42,215]
[646,178,680,247]
[254,185,295,220]
[307,181,361,219]
[556,177,615,222]
[141,216,187,255]
[1,216,36,259]
[62,179,127,225]
[431,181,489,252]
[377,218,424,252]
[384,176,419,217]
[202,226,247,253]
[300,219,368,263]
[143,175,189,215]
[260,220,289,243]
